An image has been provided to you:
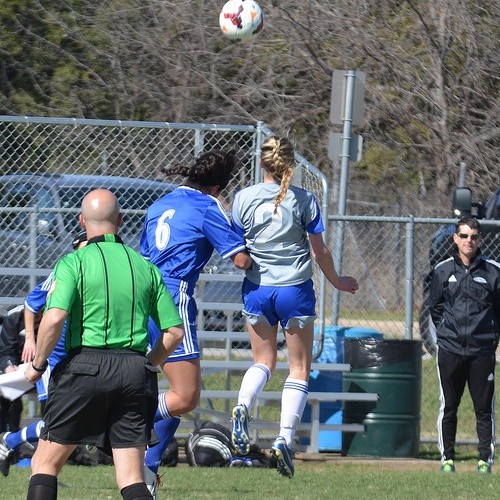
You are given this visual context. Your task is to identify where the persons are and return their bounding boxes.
[1,299,47,465]
[25,188,185,500]
[430,215,500,475]
[0,230,87,479]
[227,134,359,478]
[136,150,252,500]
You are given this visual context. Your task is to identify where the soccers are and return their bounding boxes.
[219,0,264,41]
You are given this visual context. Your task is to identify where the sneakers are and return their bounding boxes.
[0,432,14,477]
[231,404,253,454]
[271,436,295,479]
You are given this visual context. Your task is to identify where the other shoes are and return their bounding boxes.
[144,463,166,500]
[441,460,455,473]
[477,460,491,475]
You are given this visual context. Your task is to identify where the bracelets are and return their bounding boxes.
[31,357,49,372]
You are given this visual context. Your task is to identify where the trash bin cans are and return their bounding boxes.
[294,326,384,452]
[342,336,423,456]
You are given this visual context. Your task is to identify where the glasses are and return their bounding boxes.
[458,233,479,240]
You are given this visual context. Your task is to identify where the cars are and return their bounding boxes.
[0,172,286,349]
